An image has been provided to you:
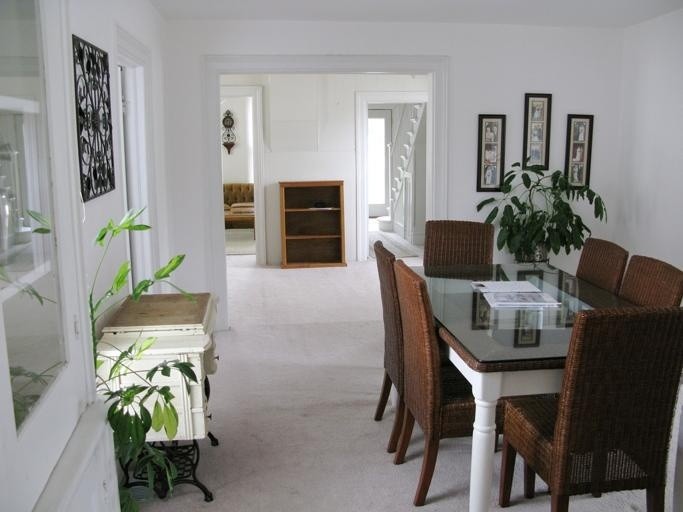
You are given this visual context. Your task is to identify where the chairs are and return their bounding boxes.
[423,219,494,264]
[497,306,682,512]
[576,236,629,296]
[370,239,470,456]
[390,258,535,509]
[617,254,682,311]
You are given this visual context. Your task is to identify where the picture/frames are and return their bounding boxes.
[555,269,583,328]
[476,112,508,192]
[562,113,595,191]
[470,291,500,331]
[521,92,553,170]
[513,268,545,347]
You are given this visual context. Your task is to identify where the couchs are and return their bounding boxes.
[223,183,253,212]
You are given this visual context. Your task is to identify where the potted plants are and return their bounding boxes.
[475,156,608,263]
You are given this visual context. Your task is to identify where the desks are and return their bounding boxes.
[224,213,254,241]
[405,263,681,511]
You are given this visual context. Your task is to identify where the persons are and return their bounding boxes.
[572,146,581,162]
[485,125,495,142]
[531,124,542,142]
[573,164,578,184]
[533,103,544,122]
[484,166,492,185]
[578,124,584,141]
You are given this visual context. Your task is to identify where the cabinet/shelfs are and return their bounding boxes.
[2,2,76,444]
[279,179,346,267]
[98,289,218,504]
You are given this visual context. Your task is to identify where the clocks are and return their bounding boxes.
[221,109,237,156]
